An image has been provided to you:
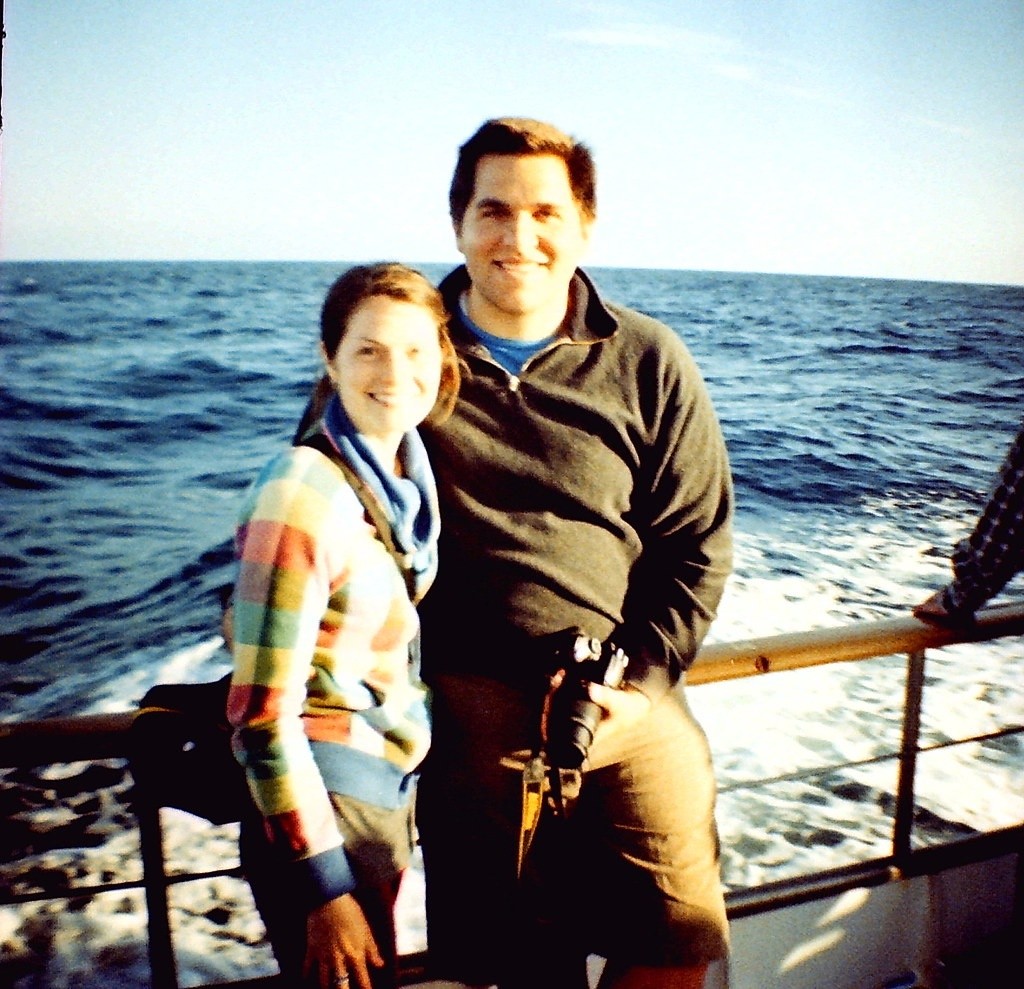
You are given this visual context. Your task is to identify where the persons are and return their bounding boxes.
[219,115,733,989]
[912,429,1024,622]
[224,261,459,989]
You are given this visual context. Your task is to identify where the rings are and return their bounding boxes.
[334,974,349,985]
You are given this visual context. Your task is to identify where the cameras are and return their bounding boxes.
[544,633,631,772]
